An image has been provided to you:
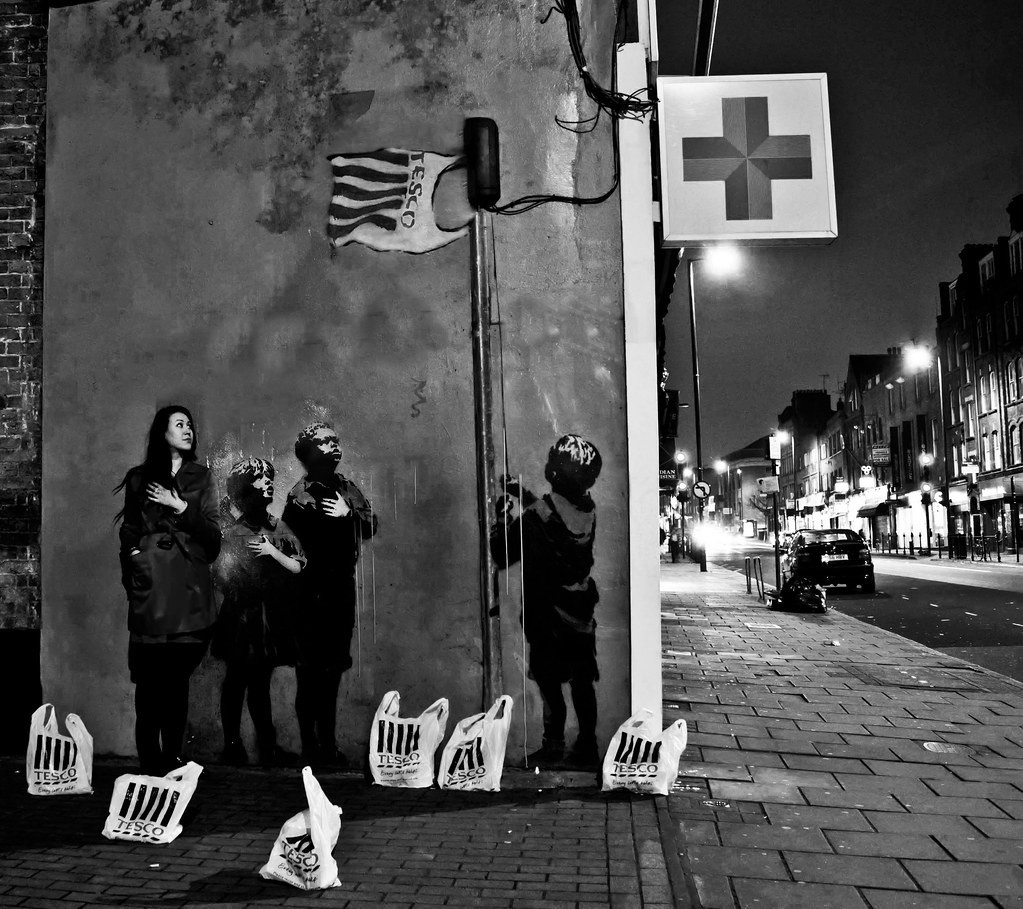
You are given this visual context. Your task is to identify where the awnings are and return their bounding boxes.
[856,502,887,518]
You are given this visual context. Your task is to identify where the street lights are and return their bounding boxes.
[712,459,733,534]
[904,344,955,559]
[774,430,799,530]
[683,241,743,544]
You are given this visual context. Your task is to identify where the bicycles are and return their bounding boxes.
[971,537,992,563]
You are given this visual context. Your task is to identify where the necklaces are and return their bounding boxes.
[172,471,175,474]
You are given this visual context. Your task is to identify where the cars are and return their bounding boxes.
[777,528,876,595]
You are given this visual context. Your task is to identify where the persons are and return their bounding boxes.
[114,406,220,776]
[859,529,867,542]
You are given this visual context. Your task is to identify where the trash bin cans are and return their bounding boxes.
[953,534,968,560]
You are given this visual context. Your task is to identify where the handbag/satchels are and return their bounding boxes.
[437,696,512,793]
[27,703,95,796]
[367,690,451,789]
[599,711,688,799]
[260,763,344,894]
[101,761,205,848]
